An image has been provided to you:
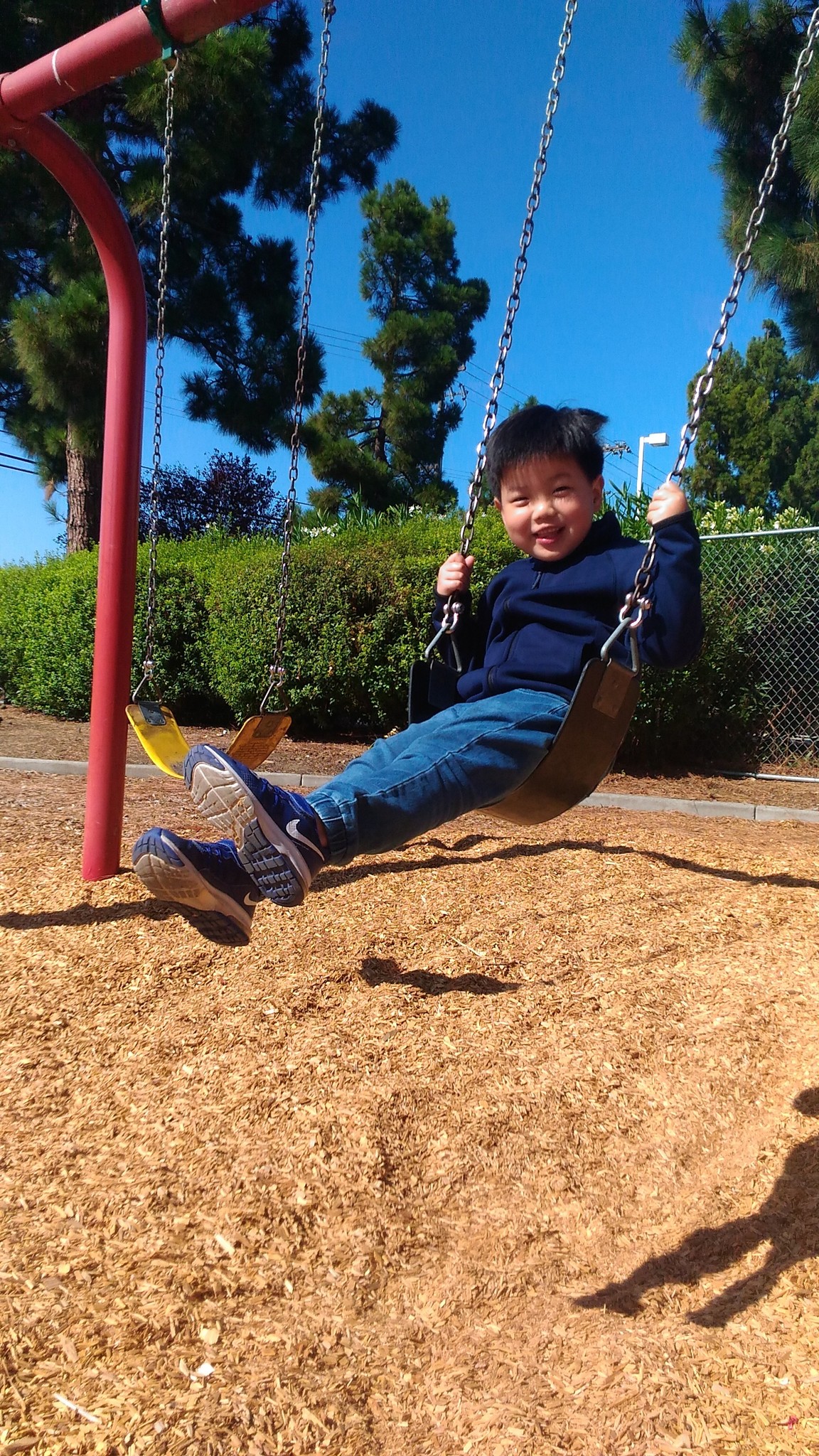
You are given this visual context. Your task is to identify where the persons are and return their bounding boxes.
[129,405,702,949]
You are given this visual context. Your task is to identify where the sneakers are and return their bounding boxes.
[180,743,329,906]
[132,826,266,946]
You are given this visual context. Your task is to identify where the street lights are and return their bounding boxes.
[635,432,670,522]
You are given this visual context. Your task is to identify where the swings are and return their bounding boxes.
[121,1,338,790]
[383,1,819,827]
